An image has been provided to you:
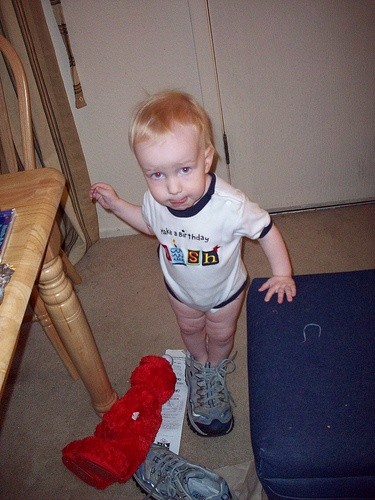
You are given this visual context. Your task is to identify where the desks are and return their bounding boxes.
[0,168,119,419]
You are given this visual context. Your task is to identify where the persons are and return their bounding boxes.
[89,90,296,365]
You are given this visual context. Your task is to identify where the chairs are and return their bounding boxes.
[0,34,81,380]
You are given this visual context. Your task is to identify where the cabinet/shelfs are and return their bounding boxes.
[40,0,375,239]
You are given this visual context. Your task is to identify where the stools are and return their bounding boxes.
[247,269,375,500]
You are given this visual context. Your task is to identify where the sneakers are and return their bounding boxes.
[132,442,232,500]
[185,341,237,437]
